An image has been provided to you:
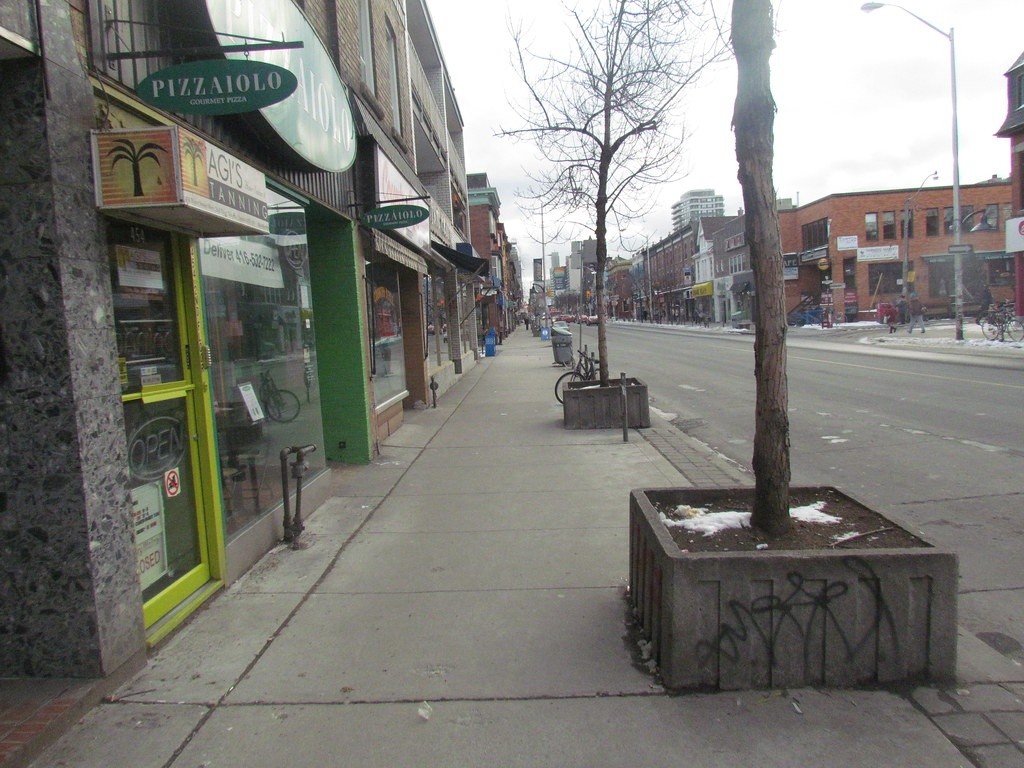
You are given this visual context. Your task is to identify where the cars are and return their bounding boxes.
[586,316,598,326]
[428,321,447,342]
[575,315,588,324]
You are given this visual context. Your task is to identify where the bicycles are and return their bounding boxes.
[553,349,601,404]
[981,302,1023,343]
[259,369,301,424]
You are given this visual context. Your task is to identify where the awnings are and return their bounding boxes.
[426,240,493,329]
[691,282,713,297]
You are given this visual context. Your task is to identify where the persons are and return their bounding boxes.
[525,317,529,330]
[643,310,647,321]
[884,292,926,334]
[975,285,993,325]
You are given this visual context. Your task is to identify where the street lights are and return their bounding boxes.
[541,187,583,328]
[860,1,964,341]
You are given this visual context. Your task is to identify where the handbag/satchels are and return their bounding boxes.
[921,306,927,313]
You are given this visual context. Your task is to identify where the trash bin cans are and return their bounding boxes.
[730,311,744,329]
[550,327,572,364]
[485,334,496,357]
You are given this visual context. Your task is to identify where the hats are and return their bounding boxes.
[911,292,917,297]
